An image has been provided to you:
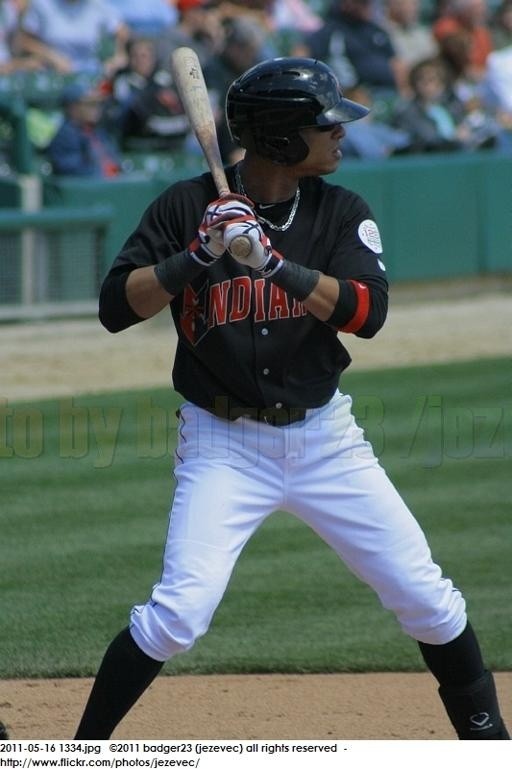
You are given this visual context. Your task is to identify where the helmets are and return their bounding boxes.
[223,55,373,168]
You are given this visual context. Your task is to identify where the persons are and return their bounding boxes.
[71,54,512,740]
[0,0,512,178]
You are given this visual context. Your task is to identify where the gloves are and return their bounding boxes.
[185,190,259,269]
[216,211,287,280]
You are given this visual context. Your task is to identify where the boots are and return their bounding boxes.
[435,665,510,740]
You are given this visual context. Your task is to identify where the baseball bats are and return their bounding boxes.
[171,47,251,258]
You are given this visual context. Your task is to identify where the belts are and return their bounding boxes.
[178,380,309,430]
